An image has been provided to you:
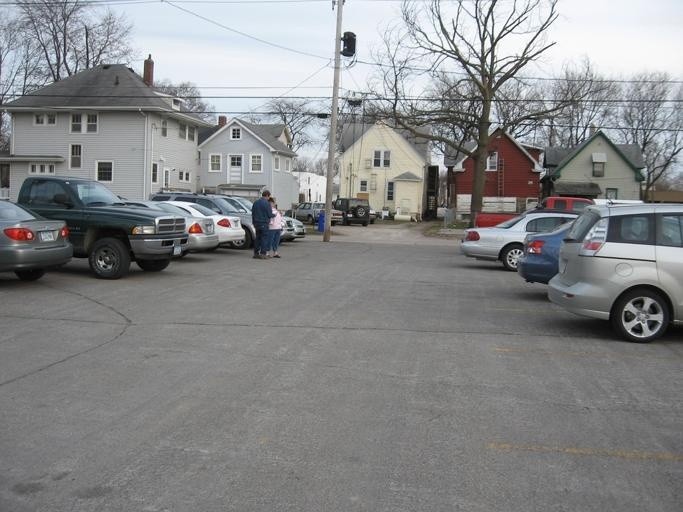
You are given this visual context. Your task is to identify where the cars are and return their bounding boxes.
[458,208,580,270]
[545,202,681,345]
[536,195,595,214]
[149,174,373,259]
[0,198,73,281]
[515,221,572,284]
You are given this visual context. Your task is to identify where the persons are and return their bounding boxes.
[266,197,284,258]
[251,189,277,260]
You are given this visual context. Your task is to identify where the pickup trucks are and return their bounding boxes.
[0,172,186,278]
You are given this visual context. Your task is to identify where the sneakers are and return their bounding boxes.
[261,254,268,259]
[253,255,264,259]
[273,255,280,258]
[266,255,271,258]
